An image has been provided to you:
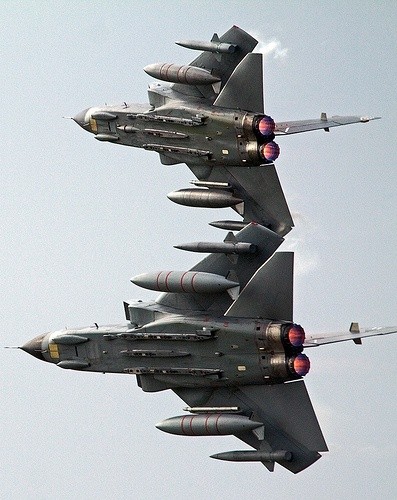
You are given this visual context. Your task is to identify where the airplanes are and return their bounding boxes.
[3,222,394,477]
[64,22,384,235]
